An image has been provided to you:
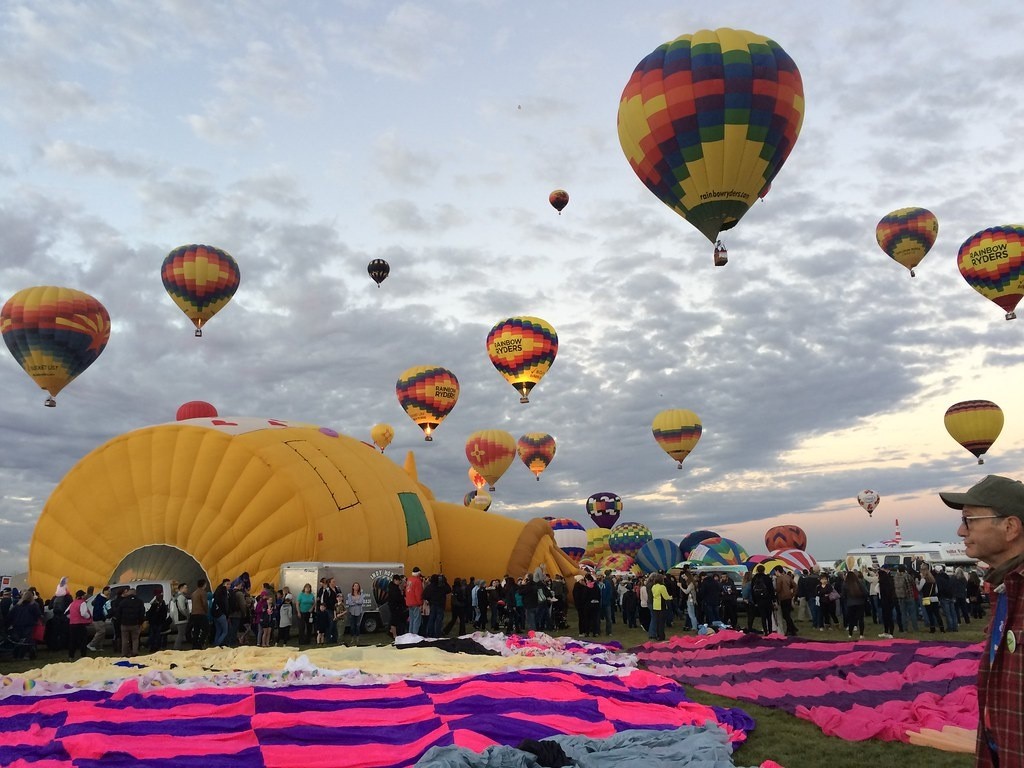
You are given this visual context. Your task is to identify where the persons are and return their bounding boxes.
[939,474,1023,768]
[0,554,996,654]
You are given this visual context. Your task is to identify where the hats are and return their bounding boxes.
[413,567,421,572]
[935,566,942,573]
[260,591,268,597]
[285,594,292,599]
[939,475,1024,520]
[684,564,690,567]
[3,590,11,594]
[454,577,461,581]
[574,575,584,582]
[153,588,161,593]
[76,590,86,596]
[625,583,633,591]
[336,593,342,596]
[320,577,329,582]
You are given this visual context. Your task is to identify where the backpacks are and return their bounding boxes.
[752,576,769,600]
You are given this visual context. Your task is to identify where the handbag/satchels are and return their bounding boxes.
[178,609,187,621]
[829,589,840,600]
[32,620,45,640]
[922,598,930,605]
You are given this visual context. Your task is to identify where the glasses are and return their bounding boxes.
[3,594,9,596]
[962,516,1009,529]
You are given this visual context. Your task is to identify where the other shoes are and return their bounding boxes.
[609,634,612,637]
[848,634,851,638]
[813,627,818,630]
[97,649,104,651]
[846,626,849,630]
[878,633,893,639]
[595,634,598,636]
[940,627,946,633]
[261,642,270,646]
[579,633,590,637]
[833,625,840,631]
[860,635,864,638]
[87,644,96,651]
[641,624,649,632]
[820,627,823,631]
[825,624,834,631]
[929,626,936,633]
[853,626,857,631]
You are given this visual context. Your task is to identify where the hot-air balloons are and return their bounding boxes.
[586,527,612,562]
[175,400,219,422]
[765,524,807,554]
[857,489,881,517]
[652,408,704,472]
[585,530,792,582]
[395,364,459,443]
[875,207,939,279]
[28,417,587,633]
[956,223,1024,321]
[485,315,560,404]
[616,26,805,267]
[0,286,112,408]
[367,258,390,286]
[943,400,1004,466]
[610,521,653,558]
[771,548,817,575]
[585,491,623,529]
[549,189,569,215]
[160,243,242,338]
